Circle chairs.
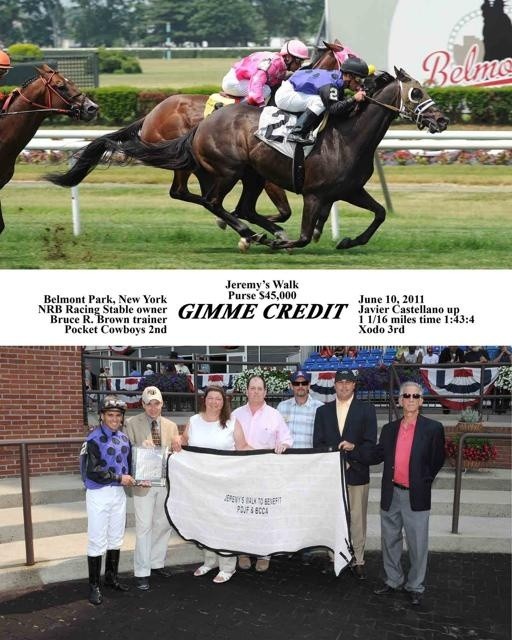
[371,348,383,355]
[383,354,394,362]
[431,346,442,352]
[342,356,355,364]
[311,365,323,370]
[303,365,312,371]
[311,350,320,359]
[369,355,381,362]
[386,347,396,355]
[329,357,340,363]
[338,363,350,371]
[363,363,376,368]
[324,365,337,370]
[358,349,370,356]
[351,364,359,369]
[356,356,367,362]
[317,358,328,364]
[305,357,315,364]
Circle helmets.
[339,56,369,78]
[97,392,127,412]
[0,51,12,68]
[279,39,309,60]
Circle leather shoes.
[134,576,150,590]
[353,564,368,579]
[151,567,173,579]
[373,581,398,595]
[402,586,422,606]
[238,557,252,569]
[255,558,270,571]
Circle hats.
[334,368,355,383]
[290,370,310,381]
[141,385,163,404]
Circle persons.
[129,365,139,374]
[278,370,327,573]
[104,367,110,378]
[145,364,154,375]
[418,348,438,367]
[226,376,292,573]
[346,348,357,356]
[96,367,105,374]
[466,347,490,364]
[179,387,247,583]
[79,398,150,605]
[338,382,446,607]
[1,53,13,110]
[217,39,311,107]
[320,347,334,357]
[439,346,463,415]
[274,56,367,146]
[129,385,182,591]
[176,357,190,373]
[193,357,210,373]
[315,368,377,578]
[493,347,512,412]
[394,347,422,368]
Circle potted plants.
[457,407,482,434]
[446,436,499,472]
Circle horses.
[104,64,451,256]
[39,38,378,243]
[0,64,100,236]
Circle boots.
[286,107,319,145]
[88,555,104,605]
[105,548,130,592]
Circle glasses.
[291,380,310,386]
[400,393,421,399]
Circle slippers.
[212,569,236,583]
[193,563,212,576]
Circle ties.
[151,420,161,447]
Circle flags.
[163,443,354,576]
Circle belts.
[392,481,410,491]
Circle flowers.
[234,366,293,396]
[494,365,512,393]
